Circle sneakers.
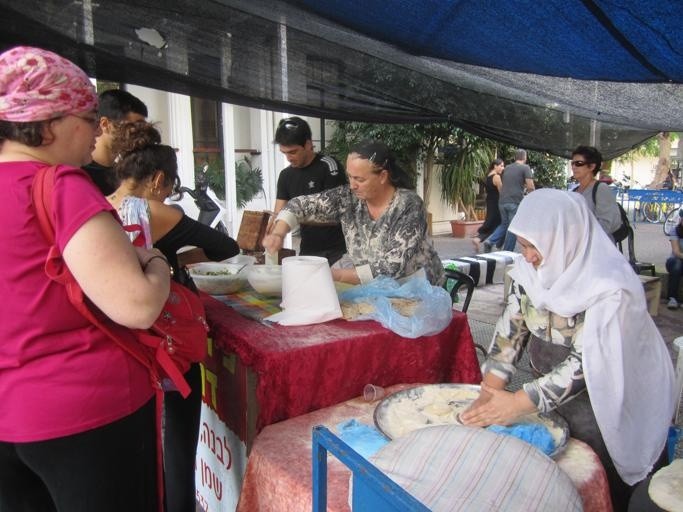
[666,295,679,309]
[471,236,481,254]
[483,239,492,253]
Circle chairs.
[443,268,488,358]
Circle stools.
[613,226,656,276]
[638,274,661,316]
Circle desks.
[235,381,612,512]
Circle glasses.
[278,116,310,139]
[570,159,590,168]
[69,112,103,128]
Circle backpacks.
[34,164,208,399]
[573,180,634,250]
[104,121,210,511]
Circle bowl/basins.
[245,264,283,298]
[186,261,248,294]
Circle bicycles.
[644,194,679,223]
[662,208,683,236]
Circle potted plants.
[193,156,268,236]
[439,135,494,237]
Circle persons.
[81,89,148,197]
[571,146,622,248]
[0,45,171,512]
[666,209,683,309]
[263,117,348,267]
[264,140,448,288]
[483,149,535,253]
[472,159,504,253]
[461,188,680,512]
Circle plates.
[373,382,570,460]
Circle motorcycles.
[170,162,229,238]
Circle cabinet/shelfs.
[147,279,485,511]
